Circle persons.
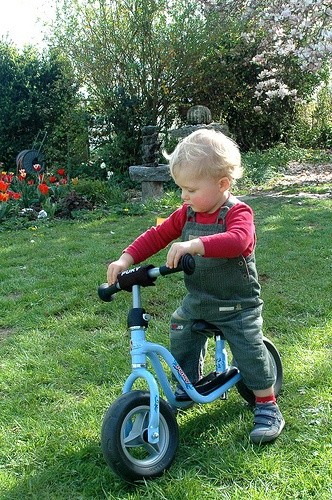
[105,127,287,443]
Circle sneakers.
[250,402,285,444]
[169,389,194,409]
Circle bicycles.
[96,252,284,485]
